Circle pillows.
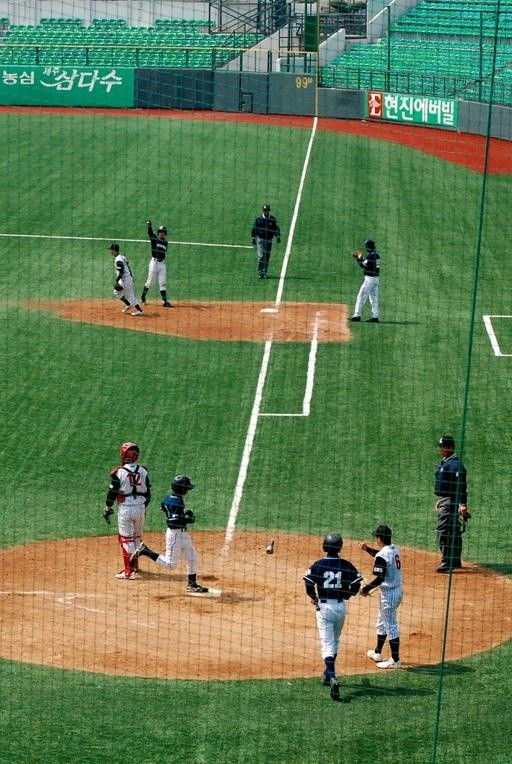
[323,532,345,553]
[121,442,140,465]
[157,225,167,235]
[365,239,375,248]
[172,475,197,490]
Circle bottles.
[107,243,120,252]
[262,204,271,210]
[438,435,455,446]
[372,525,394,538]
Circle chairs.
[367,319,380,323]
[366,650,383,659]
[322,675,329,681]
[122,305,135,314]
[186,584,208,592]
[163,302,172,306]
[436,562,452,572]
[131,311,145,316]
[454,559,461,565]
[116,572,136,578]
[264,274,270,278]
[350,316,362,322]
[131,544,146,562]
[330,678,339,700]
[257,274,263,279]
[141,298,147,306]
[376,658,398,666]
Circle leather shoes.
[252,238,258,246]
[103,509,112,526]
[276,236,282,244]
[182,514,196,523]
[185,510,193,515]
[113,282,122,290]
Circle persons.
[251,204,281,279]
[359,525,404,668]
[346,240,381,322]
[130,476,209,593]
[107,243,145,316]
[102,442,151,580]
[141,219,175,307]
[303,532,364,700]
[434,436,472,573]
